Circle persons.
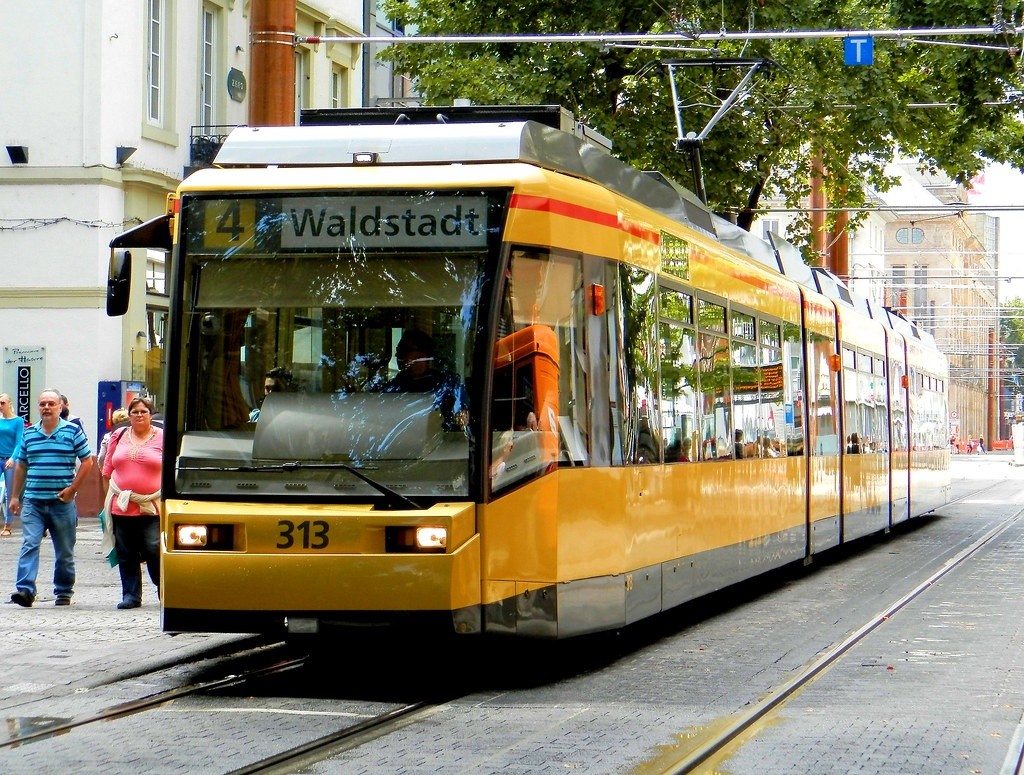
[43,395,83,537]
[948,432,1014,455]
[636,415,945,464]
[8,387,94,608]
[247,366,294,423]
[0,393,24,537]
[99,396,163,609]
[97,408,132,473]
[369,329,472,432]
[142,397,164,429]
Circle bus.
[101,55,955,643]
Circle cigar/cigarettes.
[13,506,20,514]
